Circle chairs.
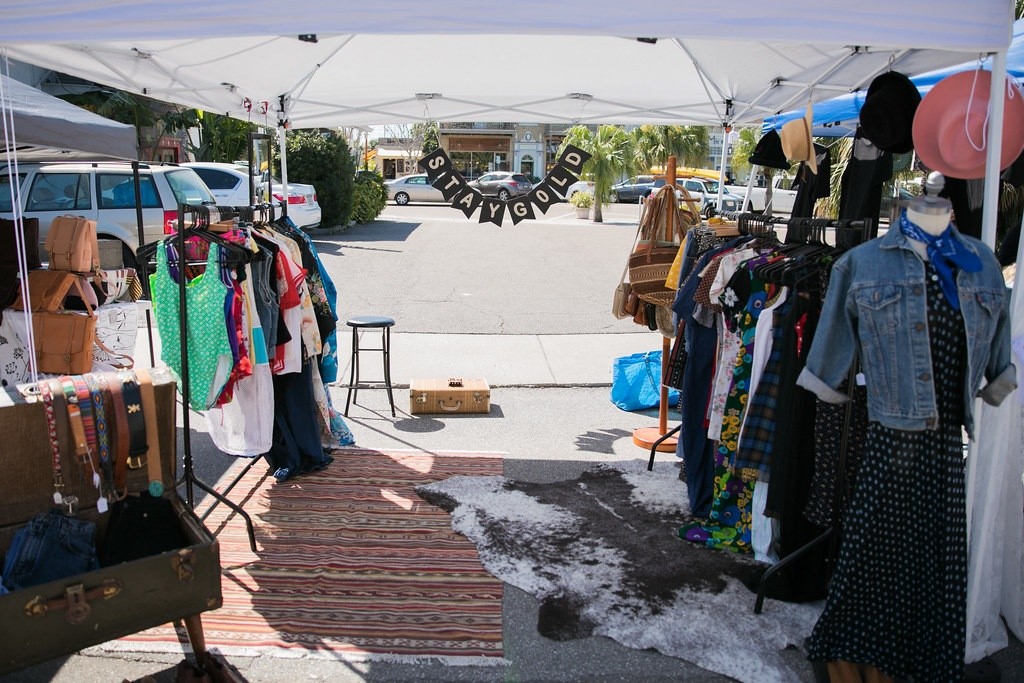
[32,188,61,211]
[64,185,85,208]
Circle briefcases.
[409,376,491,415]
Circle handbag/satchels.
[610,350,681,412]
[612,182,702,339]
[12,214,144,375]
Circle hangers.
[707,207,852,291]
[135,201,289,267]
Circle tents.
[740,9,1023,213]
[0,64,157,371]
[0,1,1017,566]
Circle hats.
[781,101,818,175]
[748,129,791,170]
[860,71,922,154]
[912,69,1024,179]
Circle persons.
[797,196,1019,683]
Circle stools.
[344,316,396,417]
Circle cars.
[879,185,915,217]
[608,175,656,203]
[384,174,482,205]
[533,174,596,198]
[102,162,322,230]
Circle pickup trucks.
[723,176,798,213]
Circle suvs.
[651,176,746,219]
[0,161,220,269]
[466,172,532,201]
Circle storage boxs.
[410,378,490,414]
[0,368,222,666]
[98,239,123,270]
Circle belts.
[31,365,164,515]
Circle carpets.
[78,445,510,666]
[416,469,826,683]
